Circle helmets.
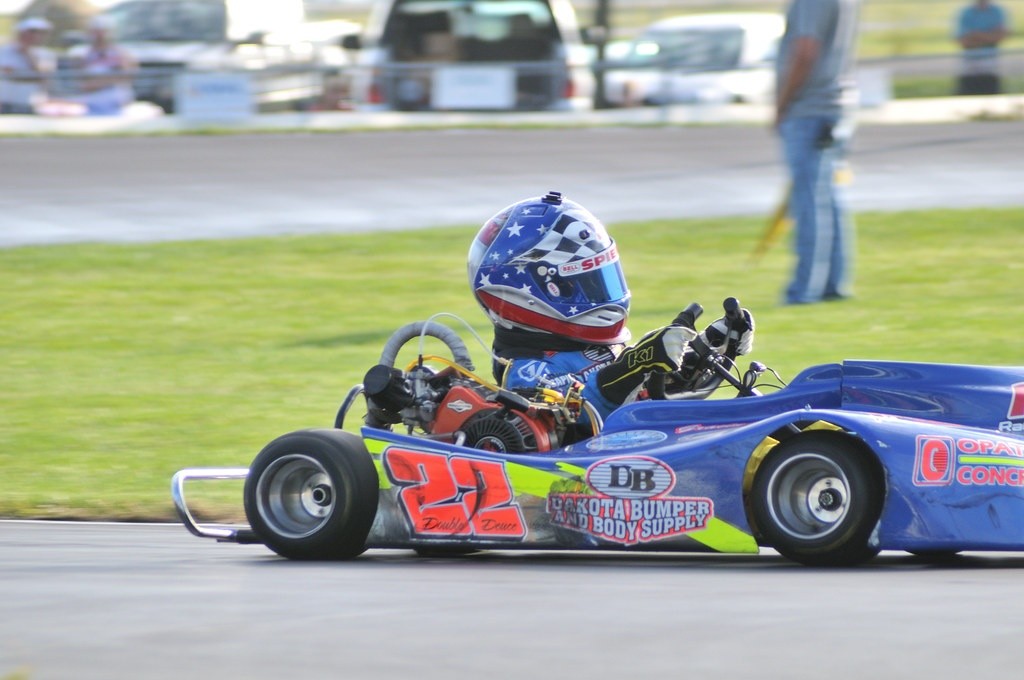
[466,191,632,346]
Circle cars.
[603,14,886,106]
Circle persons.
[772,1,864,308]
[0,16,141,120]
[952,3,1010,96]
[467,192,754,449]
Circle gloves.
[598,325,695,406]
[668,307,757,356]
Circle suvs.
[343,0,601,113]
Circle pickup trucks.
[90,2,361,114]
